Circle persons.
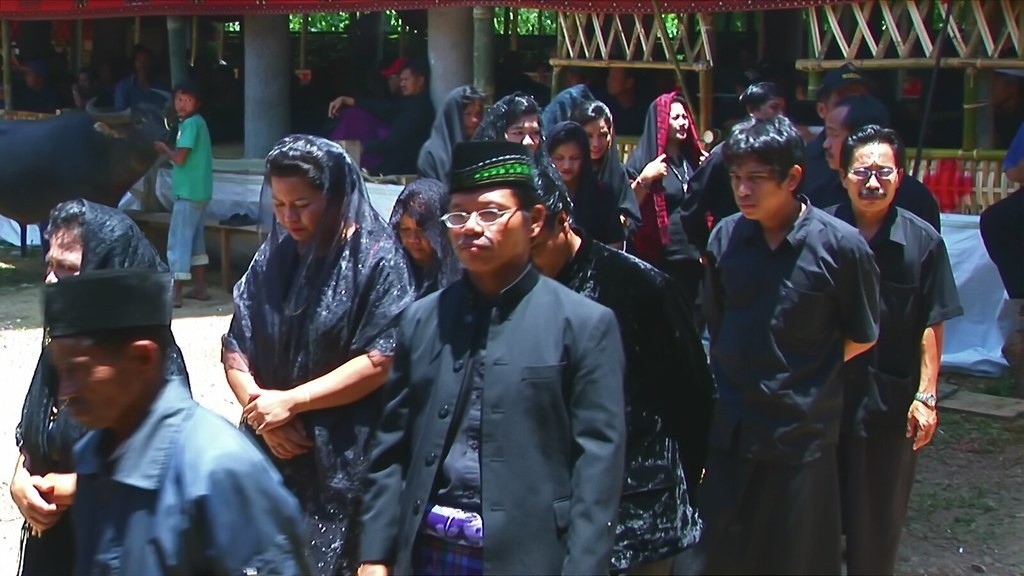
[0,17,1024,576]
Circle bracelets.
[636,177,650,194]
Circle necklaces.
[666,158,689,193]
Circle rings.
[277,446,283,451]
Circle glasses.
[438,207,518,228]
[848,167,897,180]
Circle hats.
[42,271,175,337]
[448,141,538,195]
[824,61,872,89]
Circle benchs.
[122,210,272,293]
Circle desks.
[934,211,1011,380]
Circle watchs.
[915,391,936,409]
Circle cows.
[0,88,178,259]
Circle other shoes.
[181,289,212,301]
